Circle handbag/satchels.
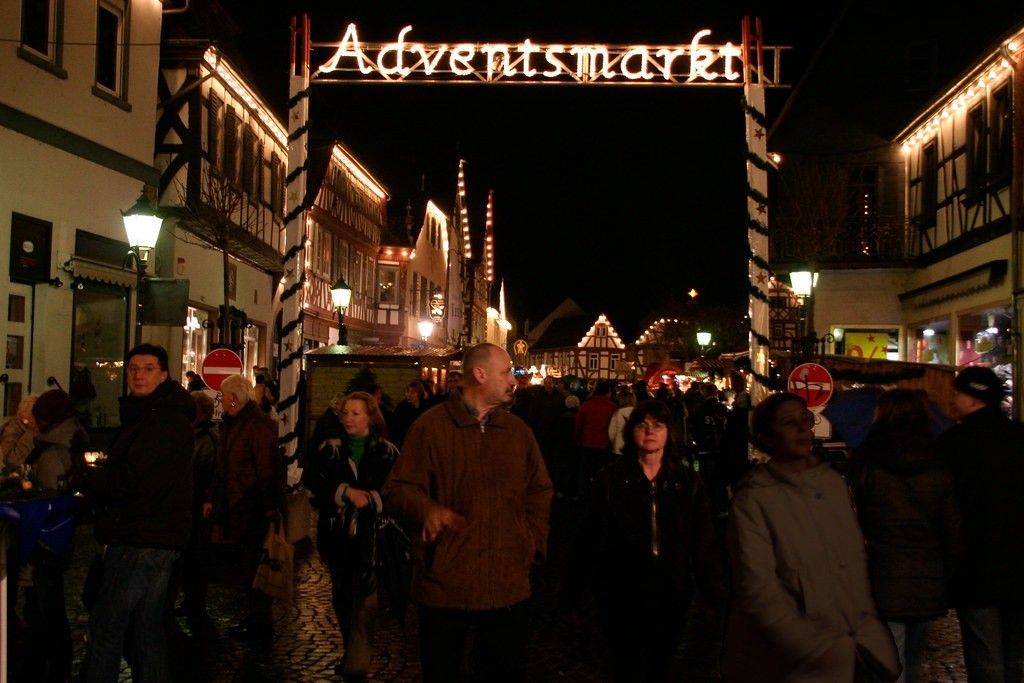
[251,512,294,603]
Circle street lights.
[789,259,820,362]
[329,276,352,346]
[119,194,165,348]
[696,326,712,372]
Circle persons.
[298,366,1024,683]
[0,367,292,683]
[729,392,903,682]
[378,342,554,683]
[76,343,196,683]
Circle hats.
[952,367,1003,406]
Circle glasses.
[634,420,666,433]
[128,365,162,374]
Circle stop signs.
[202,348,244,392]
[788,363,833,408]
